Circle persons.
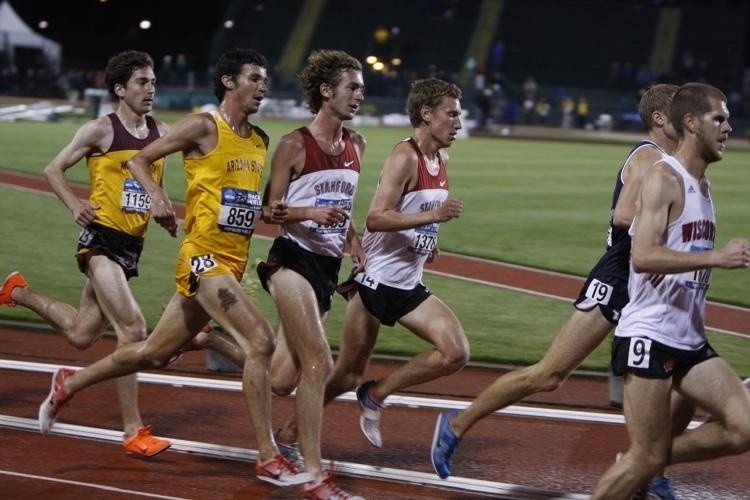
[588,84,750,500]
[428,81,709,500]
[166,45,367,500]
[0,51,175,460]
[273,77,468,473]
[35,45,316,487]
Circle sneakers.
[647,476,676,499]
[38,367,77,439]
[0,270,29,310]
[355,379,383,449]
[168,321,214,368]
[255,452,315,487]
[293,471,366,500]
[122,424,172,458]
[273,426,305,473]
[430,411,463,480]
